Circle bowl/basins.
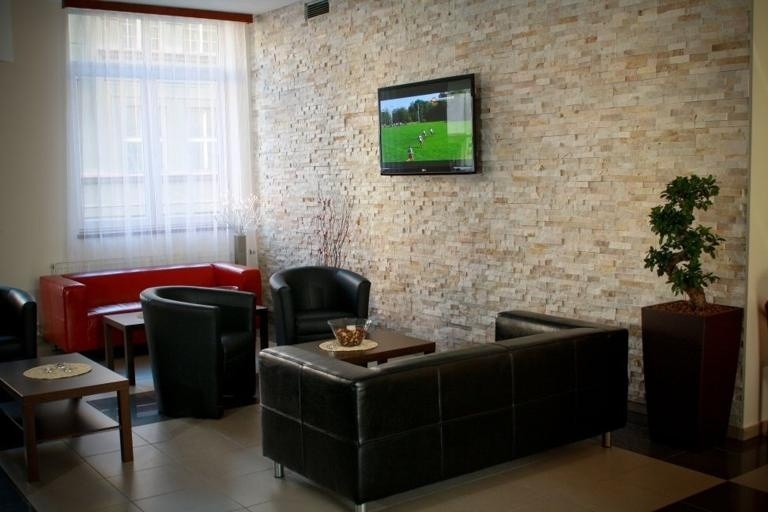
[329,318,373,347]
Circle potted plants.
[640,170,744,450]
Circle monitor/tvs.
[377,73,481,175]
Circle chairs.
[269,265,371,346]
[0,286,37,362]
[139,285,257,421]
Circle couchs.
[39,261,263,353]
[0,465,38,512]
[258,310,629,512]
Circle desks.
[289,330,436,368]
[0,352,134,483]
[102,305,269,387]
